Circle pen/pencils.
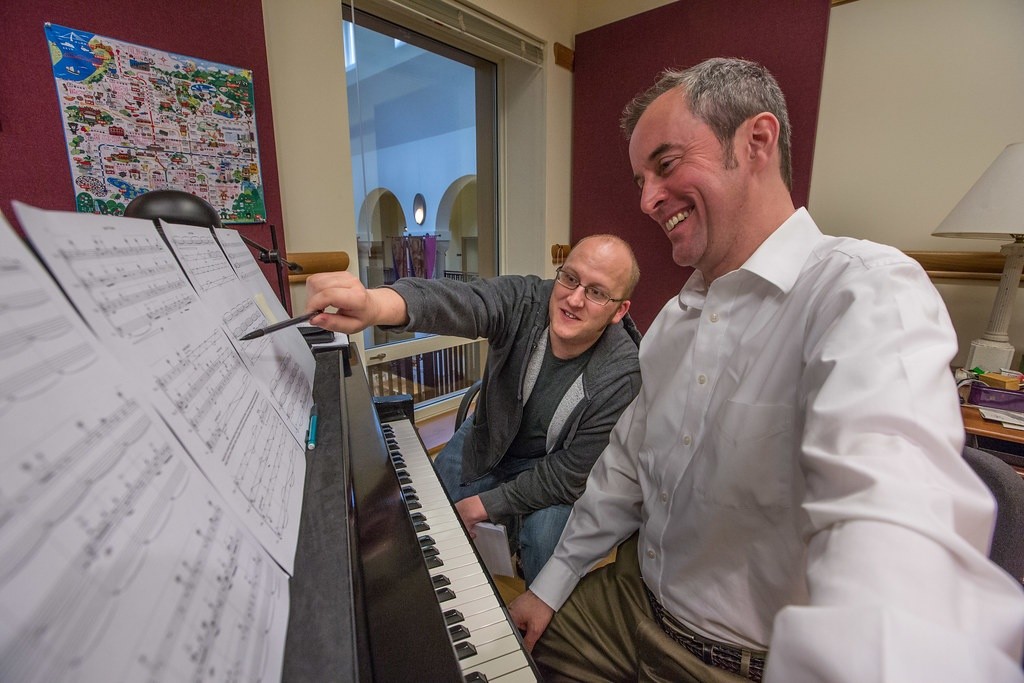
[238,309,324,342]
[304,402,319,451]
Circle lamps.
[930,143,1024,380]
[123,189,303,316]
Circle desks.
[958,386,1024,478]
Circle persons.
[306,234,643,591]
[507,57,1024,683]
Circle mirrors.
[413,193,426,225]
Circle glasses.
[556,266,626,306]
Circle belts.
[642,579,765,683]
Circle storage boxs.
[969,386,1024,413]
[980,369,1022,390]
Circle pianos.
[0,230,548,683]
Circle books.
[979,408,1024,430]
